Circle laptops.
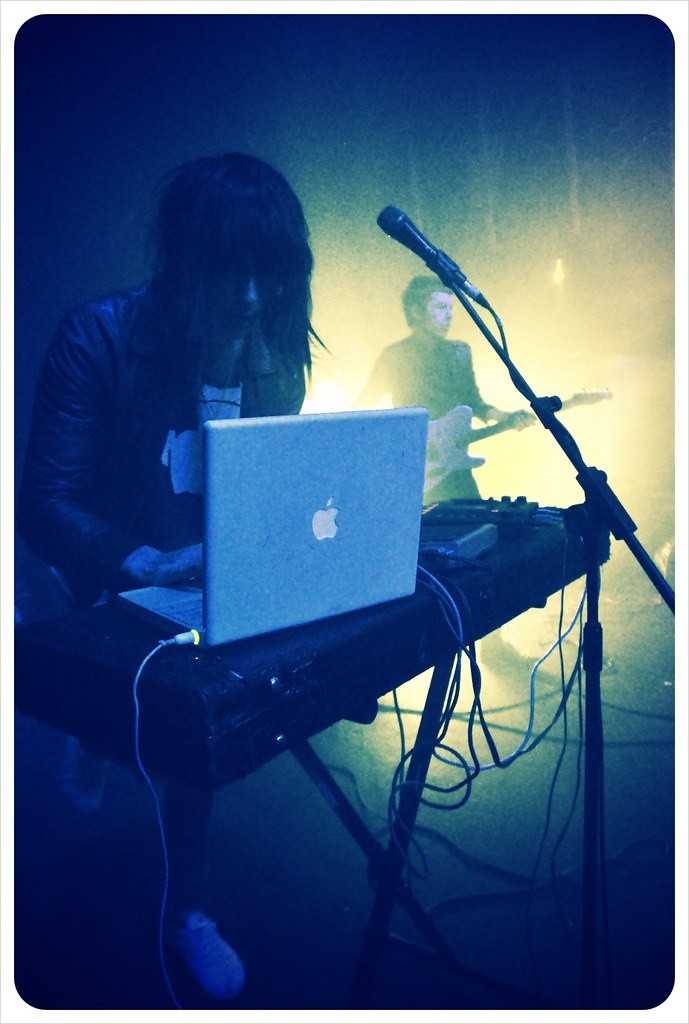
[116,407,428,649]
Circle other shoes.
[483,643,539,670]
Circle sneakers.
[178,911,244,998]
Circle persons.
[16,149,333,1003]
[364,273,533,507]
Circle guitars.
[420,392,612,493]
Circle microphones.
[377,205,490,311]
